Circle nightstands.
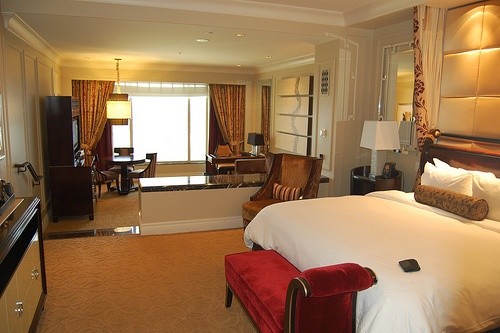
[351,165,403,196]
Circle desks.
[206,153,265,175]
[101,155,145,195]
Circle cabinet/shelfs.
[44,96,97,223]
[0,197,47,333]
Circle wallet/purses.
[399,259,420,272]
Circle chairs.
[127,153,157,191]
[109,148,134,190]
[87,155,120,198]
[241,153,324,230]
[214,143,237,175]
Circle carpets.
[34,182,260,333]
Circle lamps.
[246,133,264,156]
[360,117,400,176]
[106,58,131,125]
[398,120,410,145]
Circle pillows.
[272,183,302,202]
[414,158,500,221]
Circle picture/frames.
[397,102,413,126]
[0,94,6,159]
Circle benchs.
[225,249,377,333]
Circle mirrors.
[376,41,416,154]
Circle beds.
[241,129,500,333]
